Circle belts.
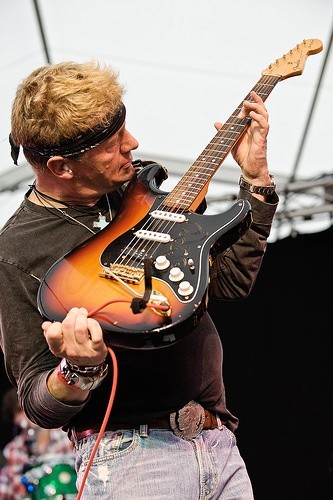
[69,398,227,445]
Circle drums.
[23,453,79,500]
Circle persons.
[0,389,71,500]
[1,63,279,500]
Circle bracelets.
[56,358,109,391]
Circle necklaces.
[29,180,112,234]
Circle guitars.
[37,37,323,349]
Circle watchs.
[238,174,277,196]
